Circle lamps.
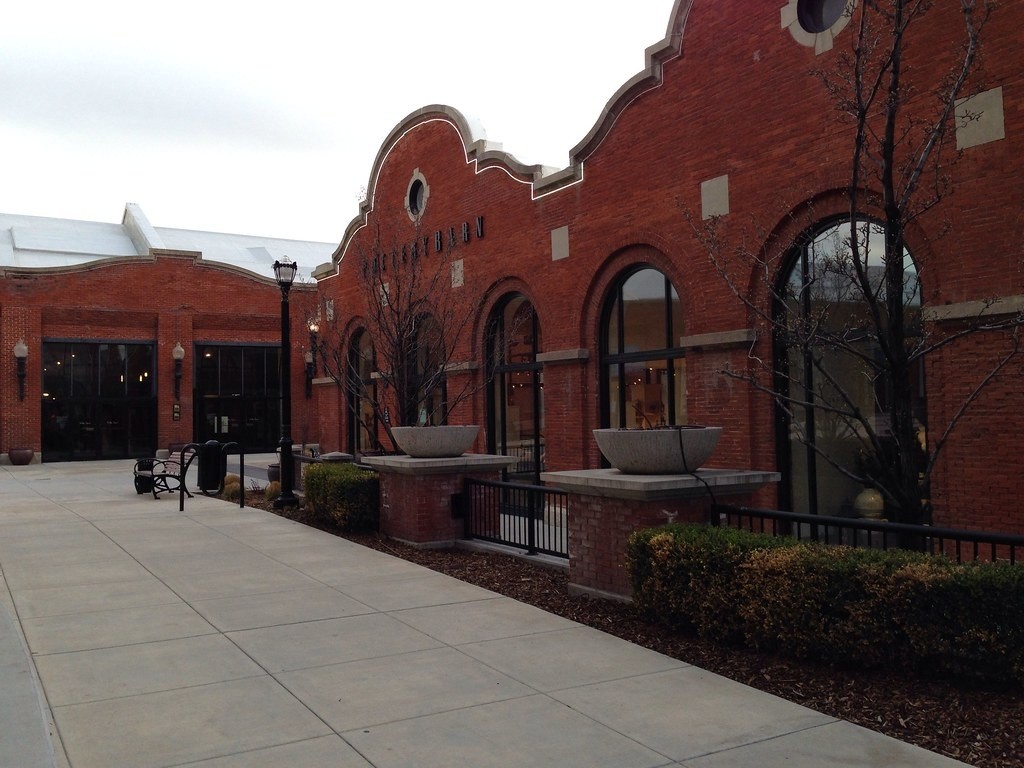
[14,338,28,401]
[305,351,314,400]
[307,317,319,375]
[172,342,185,400]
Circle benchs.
[134,451,196,499]
